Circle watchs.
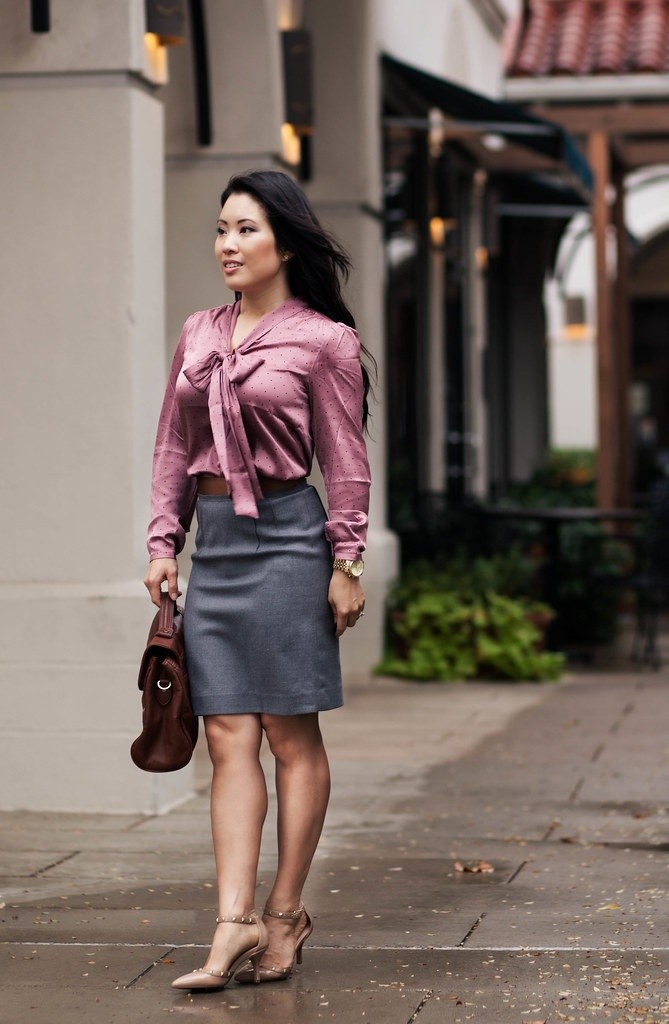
[332,559,364,578]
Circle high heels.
[171,914,269,989]
[234,900,313,985]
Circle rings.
[356,611,365,619]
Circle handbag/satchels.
[130,592,199,773]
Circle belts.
[197,475,295,497]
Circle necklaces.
[146,169,378,994]
